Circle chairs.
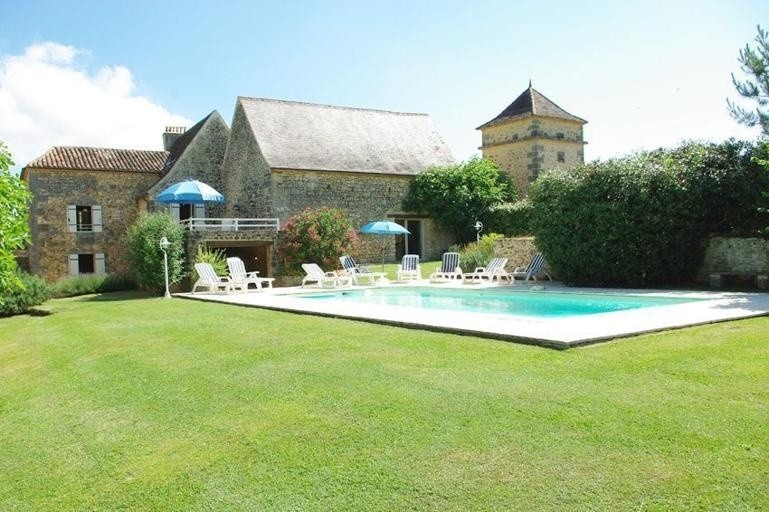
[228,256,275,293]
[300,262,351,290]
[339,255,387,287]
[191,263,236,295]
[469,257,510,284]
[397,255,421,282]
[431,252,464,281]
[508,252,553,285]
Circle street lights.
[158,236,171,298]
[473,220,484,244]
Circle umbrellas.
[356,220,411,277]
[154,180,225,231]
